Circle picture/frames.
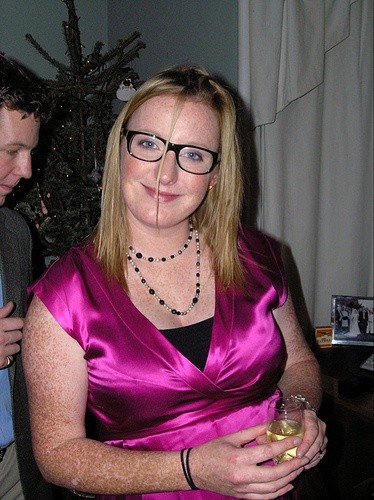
[330,295,374,345]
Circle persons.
[0,44,35,500]
[20,67,328,500]
[334,301,374,338]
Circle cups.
[265,397,305,465]
[315,326,333,348]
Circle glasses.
[120,128,221,175]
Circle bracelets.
[181,448,200,490]
[280,393,317,413]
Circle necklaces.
[126,222,202,316]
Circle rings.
[7,357,11,366]
